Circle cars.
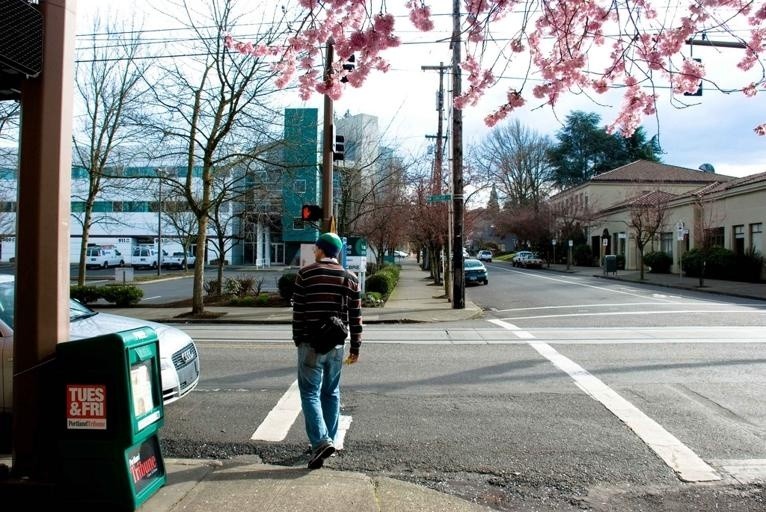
[0,274,200,413]
[463,247,493,286]
[512,251,543,268]
[384,250,408,259]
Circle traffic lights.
[302,205,322,221]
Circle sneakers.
[308,442,336,469]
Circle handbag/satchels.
[309,316,348,355]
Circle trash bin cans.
[604,255,617,276]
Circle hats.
[316,232,343,257]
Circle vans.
[131,244,168,270]
[85,245,124,270]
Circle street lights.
[462,184,492,246]
[155,168,170,276]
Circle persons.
[278,273,299,308]
[290,233,362,470]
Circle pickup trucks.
[162,251,197,270]
